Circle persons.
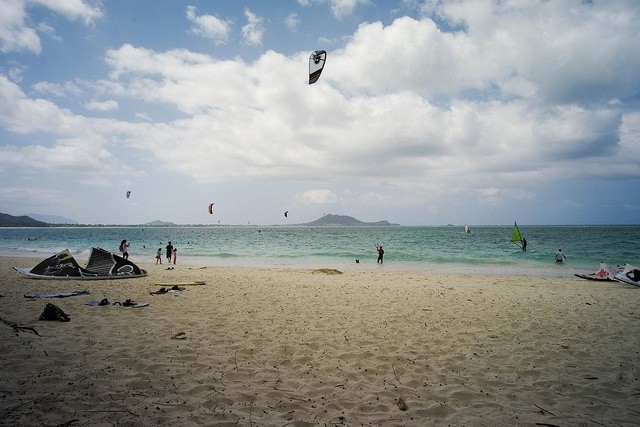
[521,237,527,251]
[173,248,177,264]
[120,239,130,260]
[375,243,385,264]
[165,241,173,262]
[155,247,164,264]
[554,248,566,263]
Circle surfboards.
[86,300,149,307]
[155,280,204,286]
[150,286,185,295]
